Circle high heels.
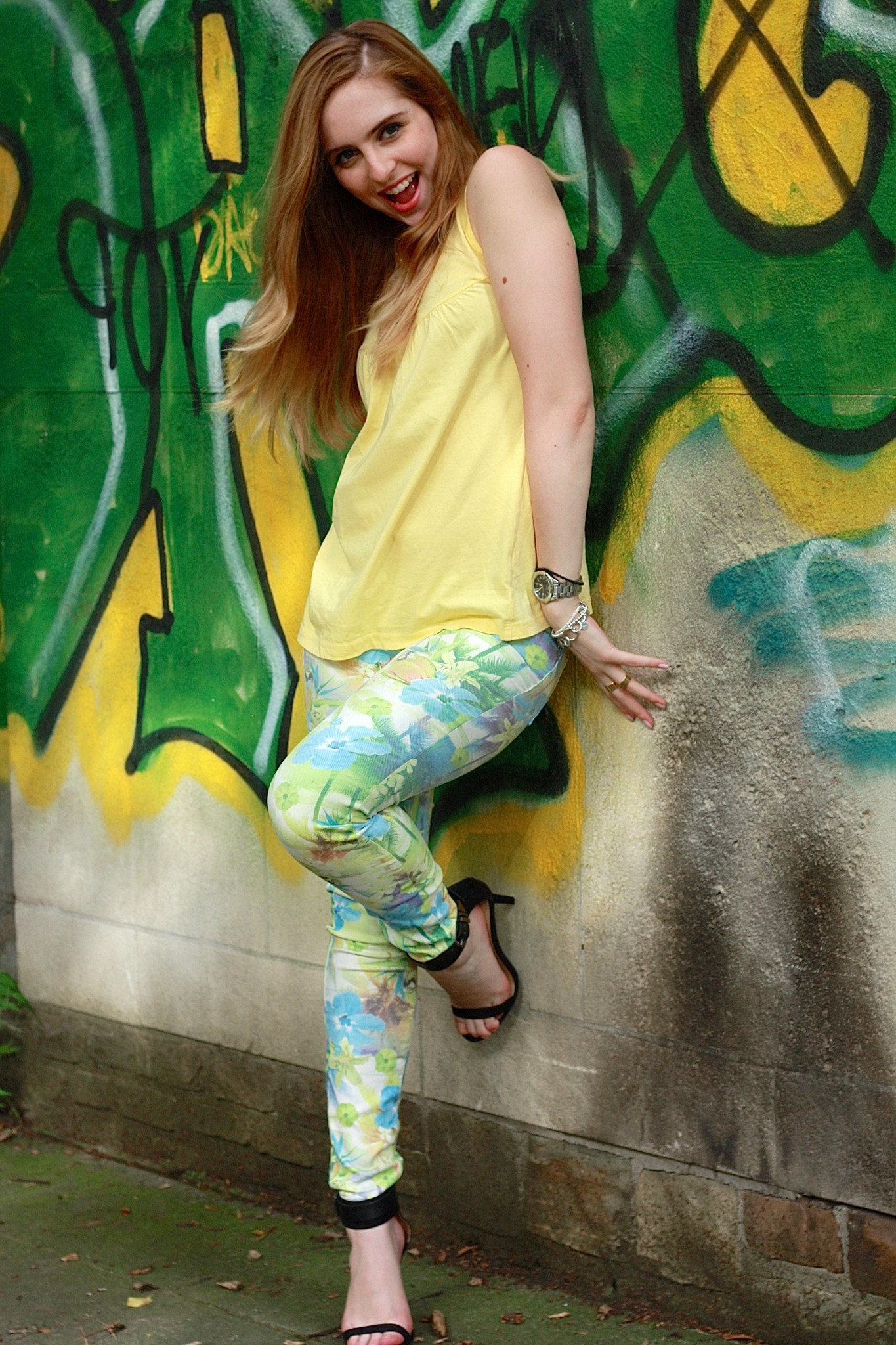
[336,1186,413,1345]
[404,877,521,1041]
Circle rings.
[604,681,618,694]
[616,671,632,690]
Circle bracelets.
[551,601,590,651]
[534,566,585,586]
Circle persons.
[210,19,670,1345]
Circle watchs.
[532,571,582,604]
